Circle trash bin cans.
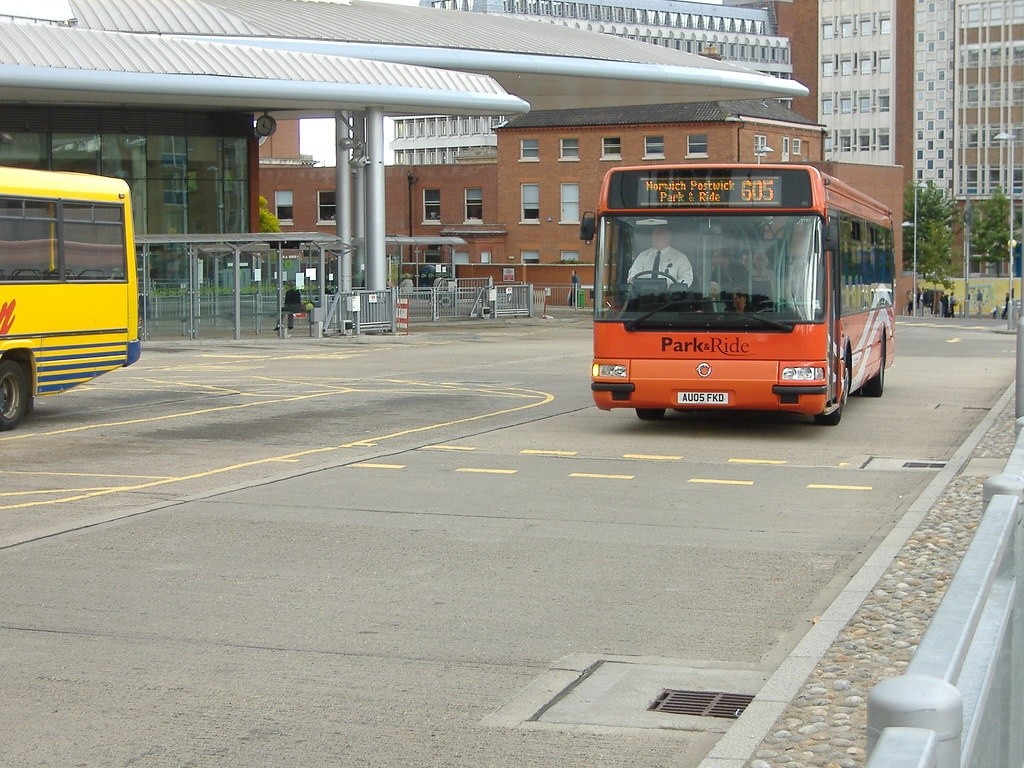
[440,278,457,307]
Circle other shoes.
[273,327,279,331]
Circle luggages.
[577,285,586,308]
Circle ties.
[652,251,661,278]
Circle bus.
[0,164,141,431]
[578,162,897,427]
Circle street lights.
[755,146,775,164]
[901,182,929,317]
[993,132,1018,331]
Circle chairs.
[748,274,771,297]
[0,268,107,281]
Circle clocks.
[256,111,277,137]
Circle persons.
[907,285,960,320]
[568,270,580,307]
[708,243,780,317]
[273,284,301,331]
[628,223,693,295]
[1000,291,1010,320]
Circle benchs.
[146,310,312,340]
